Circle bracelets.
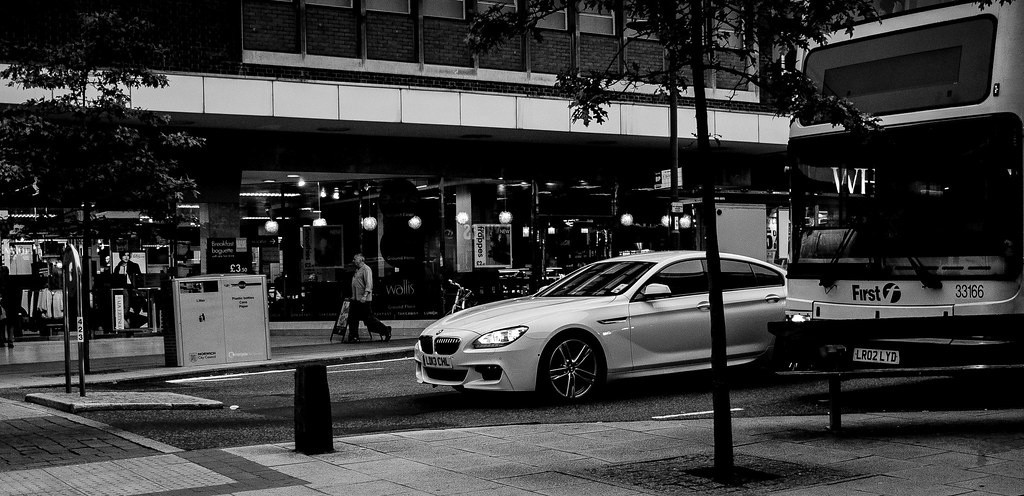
[363,295,367,297]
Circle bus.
[782,0,1023,367]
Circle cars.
[413,249,789,404]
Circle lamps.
[265,183,692,234]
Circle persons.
[490,227,508,265]
[115,252,141,274]
[343,252,391,343]
[0,265,22,347]
[315,236,336,267]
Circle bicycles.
[445,278,474,316]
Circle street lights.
[629,18,679,251]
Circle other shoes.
[0,343,6,347]
[344,336,360,343]
[8,344,14,348]
[383,326,392,341]
